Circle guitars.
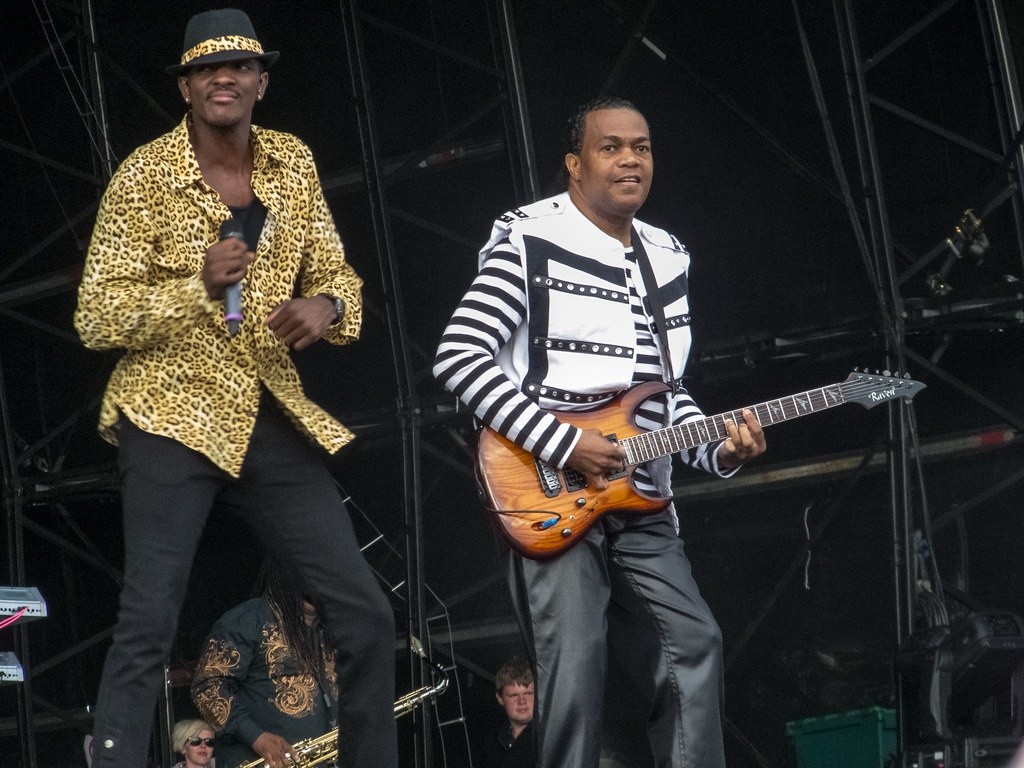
[473,364,930,564]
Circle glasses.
[184,737,215,747]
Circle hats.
[168,9,279,71]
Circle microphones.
[218,219,246,338]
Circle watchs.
[319,293,343,325]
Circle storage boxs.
[789,706,898,768]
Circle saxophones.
[229,658,453,768]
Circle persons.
[431,98,767,768]
[74,10,401,768]
[171,548,535,768]
